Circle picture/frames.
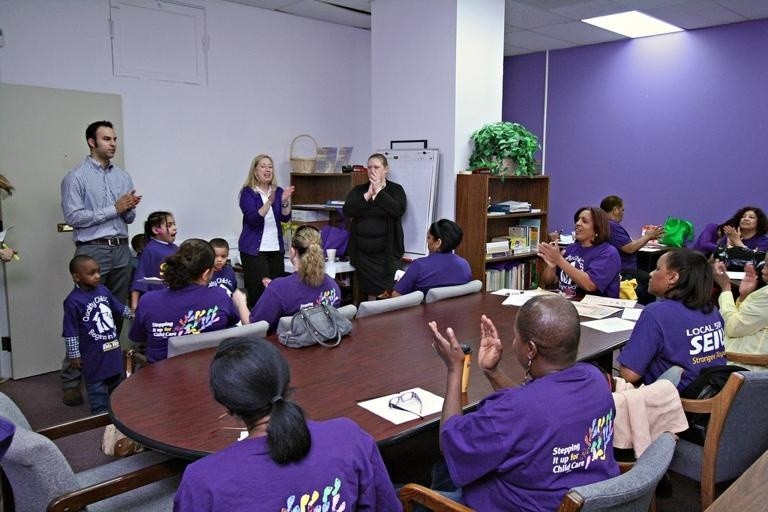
[106,0,208,85]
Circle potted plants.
[470,122,537,175]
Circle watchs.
[281,201,289,207]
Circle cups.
[326,248,336,264]
[459,344,473,393]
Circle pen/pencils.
[1,239,21,262]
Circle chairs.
[427,280,482,302]
[276,305,358,327]
[360,292,424,317]
[398,373,678,510]
[0,392,202,512]
[658,370,768,508]
[125,320,270,382]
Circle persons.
[62,255,133,415]
[600,196,666,271]
[130,233,151,309]
[238,155,295,311]
[617,249,726,393]
[206,238,237,293]
[232,227,341,337]
[61,120,142,407]
[342,153,407,301]
[172,336,404,512]
[392,220,471,297]
[127,238,241,365]
[537,206,620,299]
[128,234,145,311]
[0,173,18,262]
[394,295,621,511]
[717,207,768,259]
[691,218,730,261]
[716,254,768,370]
[131,211,179,316]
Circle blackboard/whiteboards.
[375,148,438,260]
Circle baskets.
[291,134,318,173]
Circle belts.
[78,238,129,247]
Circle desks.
[551,234,678,272]
[106,288,650,464]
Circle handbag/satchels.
[715,245,765,272]
[279,302,353,348]
[680,365,750,445]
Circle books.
[486,260,539,292]
[335,147,354,172]
[485,219,540,258]
[487,200,531,213]
[314,146,337,173]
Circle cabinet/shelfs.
[290,171,370,307]
[455,173,548,291]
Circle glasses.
[389,393,424,420]
[295,225,319,237]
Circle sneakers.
[62,387,83,405]
